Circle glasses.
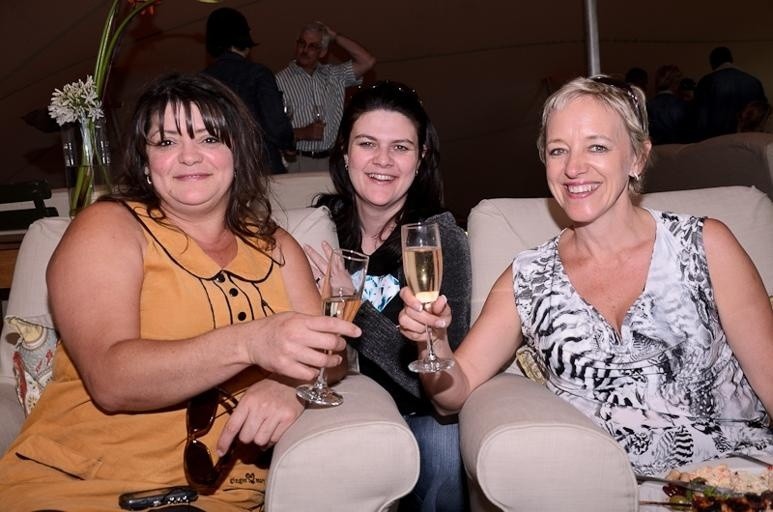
[295,38,321,51]
[589,77,644,131]
[183,385,240,486]
[369,79,424,110]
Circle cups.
[285,104,324,124]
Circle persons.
[0,60,361,511]
[625,45,772,146]
[297,85,474,511]
[199,8,296,177]
[396,72,773,479]
[273,20,376,172]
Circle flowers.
[47,0,154,214]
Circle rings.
[315,273,324,283]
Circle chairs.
[639,131,771,195]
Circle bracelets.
[331,32,342,45]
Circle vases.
[59,119,112,215]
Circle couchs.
[0,205,421,512]
[458,184,772,511]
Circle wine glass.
[401,223,456,372]
[295,247,368,406]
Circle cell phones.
[118,485,198,511]
